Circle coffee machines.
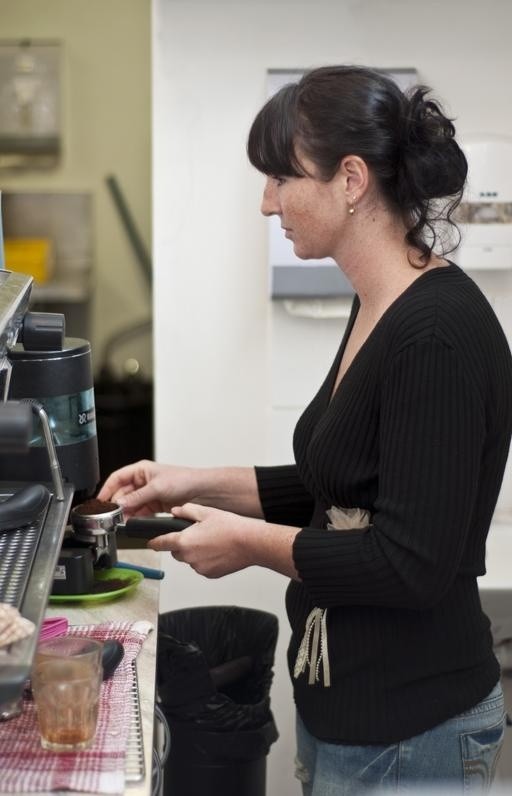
[1,265,191,722]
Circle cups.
[29,640,105,753]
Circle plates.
[49,565,145,606]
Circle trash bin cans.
[157,605,279,796]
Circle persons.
[97,65,512,796]
